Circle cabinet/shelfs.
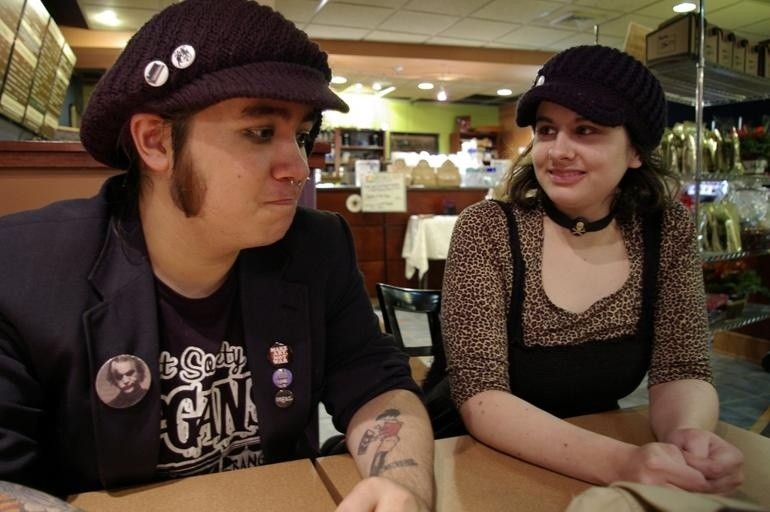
[450,130,502,159]
[643,0,770,336]
[335,127,386,178]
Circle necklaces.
[539,192,619,236]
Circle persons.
[420,45,745,496]
[0,0,440,511]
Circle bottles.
[465,166,497,187]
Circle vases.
[741,159,766,174]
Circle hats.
[513,44,670,162]
[76,0,353,171]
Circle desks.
[401,214,458,290]
[314,404,770,512]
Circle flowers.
[735,124,770,159]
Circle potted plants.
[712,269,763,320]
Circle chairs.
[375,282,442,370]
